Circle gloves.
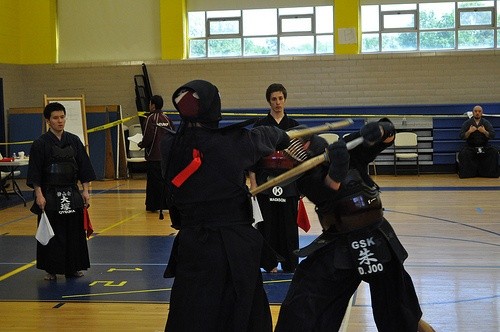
[329,140,350,176]
[360,122,382,142]
[276,129,291,151]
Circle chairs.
[393,132,419,177]
[126,140,146,181]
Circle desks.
[0,157,29,207]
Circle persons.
[457,106,500,179]
[137,95,177,212]
[274,117,435,332]
[162,80,291,332]
[26,102,97,282]
[246,83,302,273]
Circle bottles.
[12,153,16,162]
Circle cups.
[18,151,24,160]
